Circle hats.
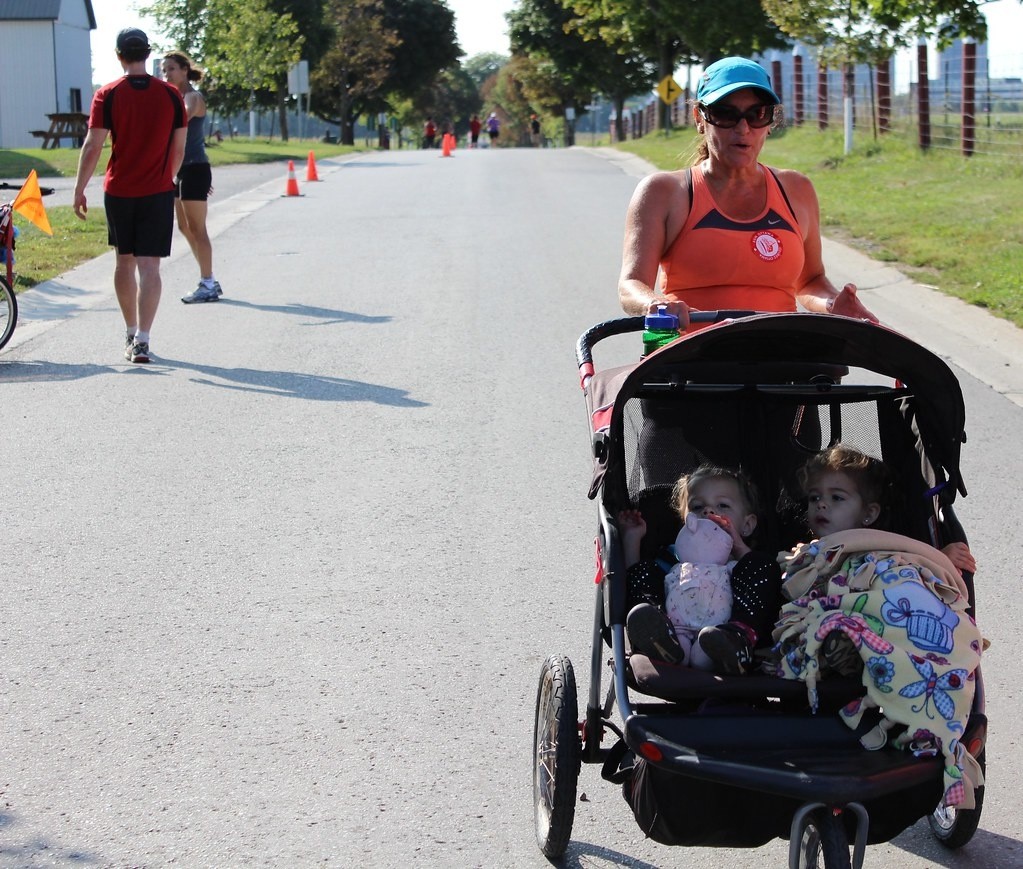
[697,57,780,105]
[117,26,149,50]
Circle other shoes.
[822,629,864,678]
[698,623,752,674]
[625,603,685,664]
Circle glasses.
[698,102,774,128]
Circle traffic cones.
[437,134,455,158]
[280,161,306,197]
[449,135,455,152]
[301,151,324,182]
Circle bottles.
[642,304,680,356]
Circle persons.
[470,116,481,148]
[426,116,437,149]
[487,112,500,148]
[161,52,223,304]
[618,468,782,687]
[617,56,879,450]
[803,444,977,751]
[529,114,540,147]
[72,27,188,363]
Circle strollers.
[532,304,989,869]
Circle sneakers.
[126,335,136,348]
[124,342,149,362]
[181,282,219,303]
[215,281,223,296]
